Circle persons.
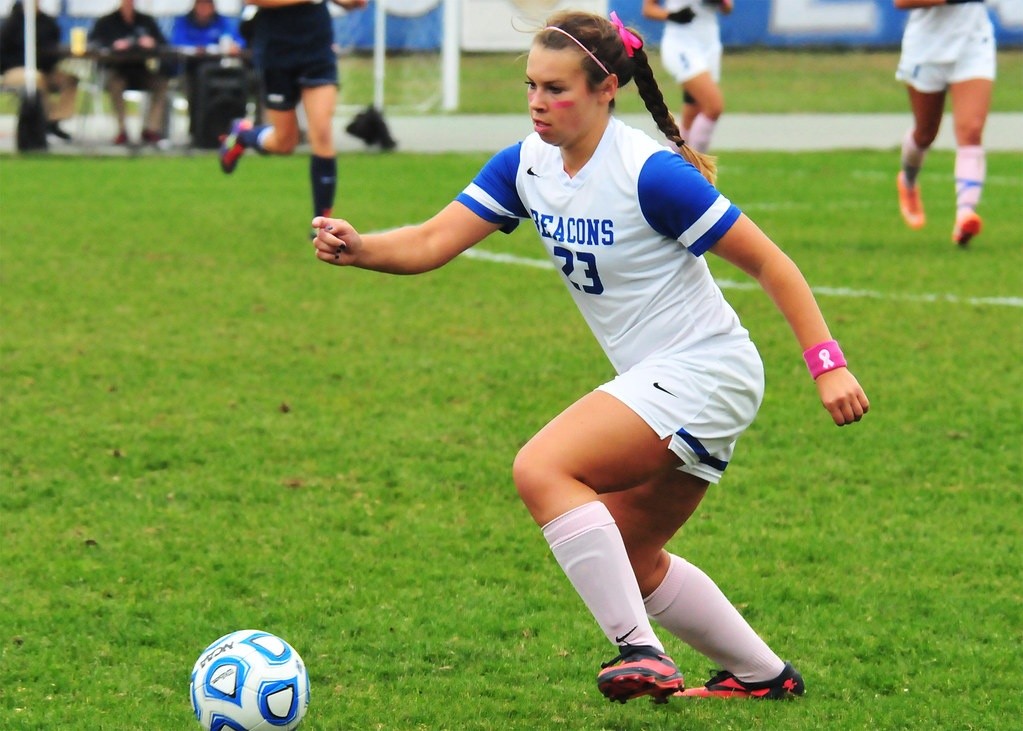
[312,10,869,705]
[0,0,367,241]
[890,0,998,247]
[642,0,733,157]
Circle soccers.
[189,629,311,731]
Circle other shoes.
[46,118,69,137]
[111,131,126,145]
[141,128,168,147]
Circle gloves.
[667,7,695,24]
[702,0,723,6]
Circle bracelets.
[802,340,847,379]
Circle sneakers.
[219,117,251,174]
[952,213,983,242]
[673,659,806,700]
[596,643,684,702]
[896,170,925,226]
